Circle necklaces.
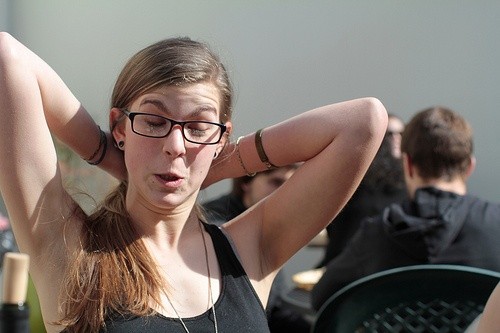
[130,216,218,333]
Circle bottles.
[0,301,30,333]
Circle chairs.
[309,265,500,333]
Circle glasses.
[119,108,226,145]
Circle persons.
[0,31,389,333]
[198,160,313,333]
[387,113,406,160]
[309,106,500,333]
[286,137,410,302]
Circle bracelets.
[235,135,257,177]
[255,127,281,169]
[83,125,107,166]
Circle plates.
[292,270,323,291]
